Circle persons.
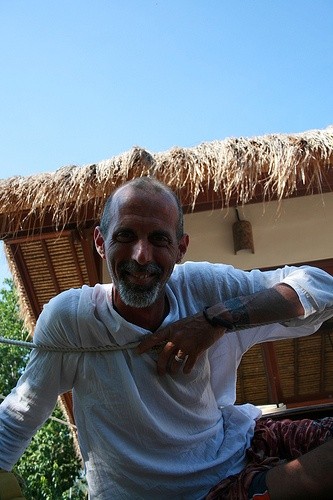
[0,172,332,500]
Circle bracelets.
[201,305,235,332]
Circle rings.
[174,354,185,363]
